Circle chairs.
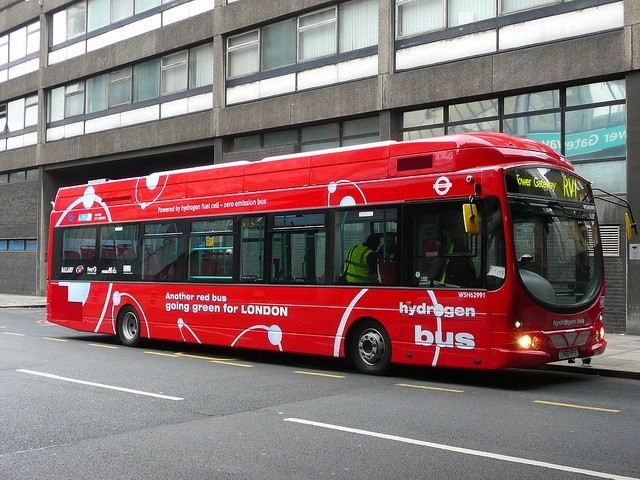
[80,245,96,260]
[101,245,116,260]
[62,251,80,260]
[117,244,134,259]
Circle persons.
[343,234,380,283]
[377,232,400,283]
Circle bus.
[46,132,637,375]
